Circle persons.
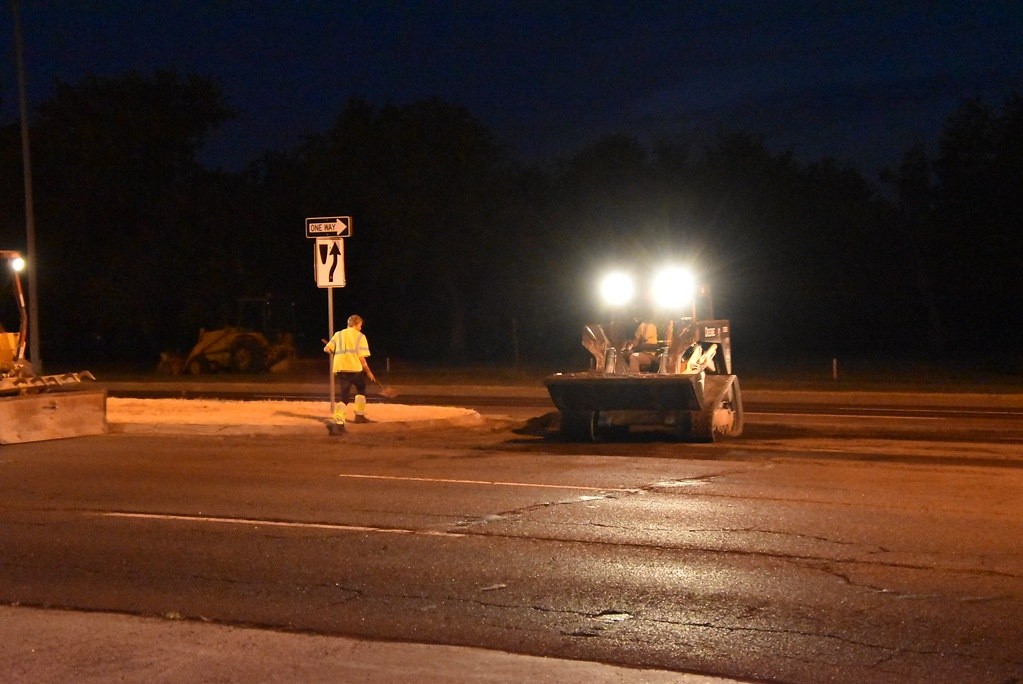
[323,315,375,436]
[621,307,658,371]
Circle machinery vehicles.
[0,249,108,445]
[157,297,298,380]
[542,266,741,442]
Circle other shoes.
[355,415,370,424]
[333,424,349,434]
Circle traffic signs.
[306,216,351,238]
